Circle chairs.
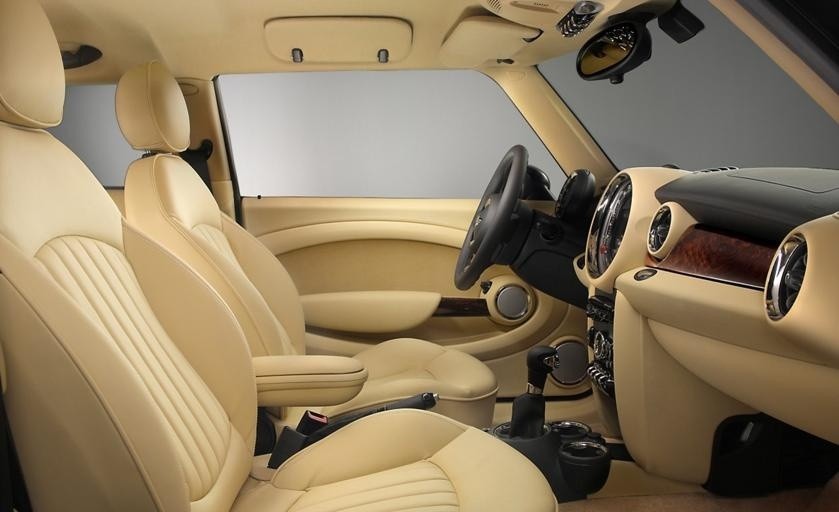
[114,61,499,434]
[0,2,562,510]
